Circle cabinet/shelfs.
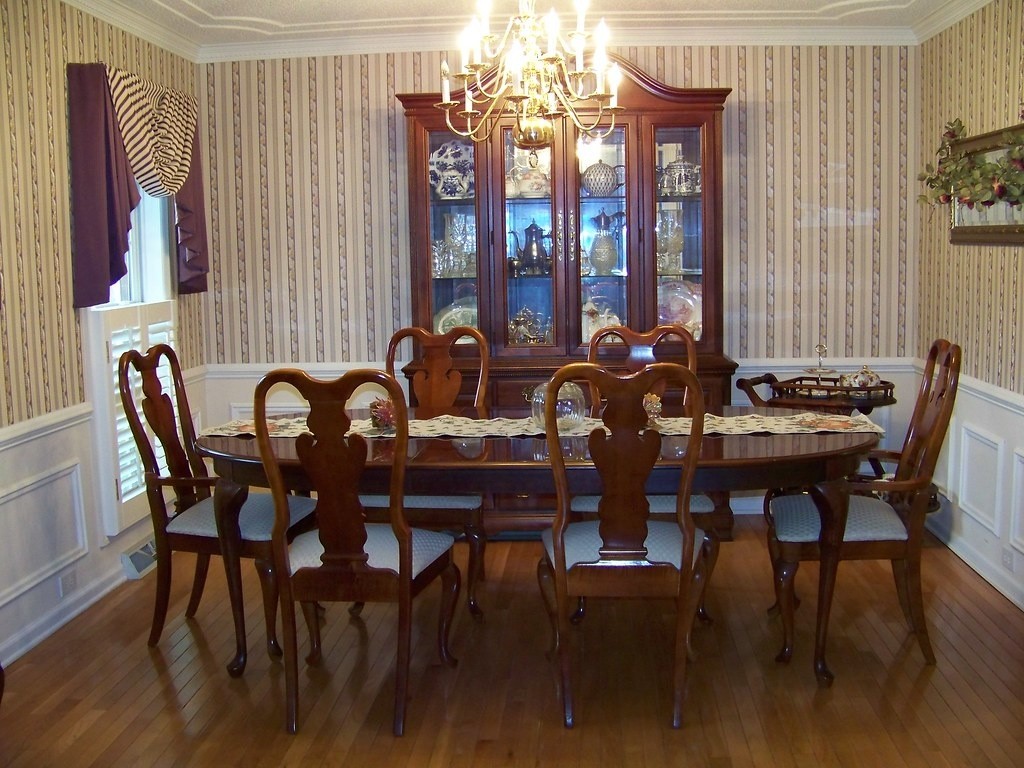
[393,47,739,542]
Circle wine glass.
[445,214,466,275]
[656,209,685,272]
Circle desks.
[191,407,883,690]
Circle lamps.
[434,1,628,169]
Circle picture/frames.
[947,122,1024,246]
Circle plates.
[657,280,702,341]
[428,139,475,199]
[434,296,477,344]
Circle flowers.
[915,119,1024,213]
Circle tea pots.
[510,166,551,198]
[666,155,702,194]
[580,159,626,196]
[505,172,520,197]
[677,177,678,177]
[588,207,617,276]
[509,218,553,275]
[582,298,609,343]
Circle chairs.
[117,341,317,680]
[567,324,723,627]
[345,325,491,620]
[762,339,962,665]
[534,362,723,730]
[253,368,463,740]
